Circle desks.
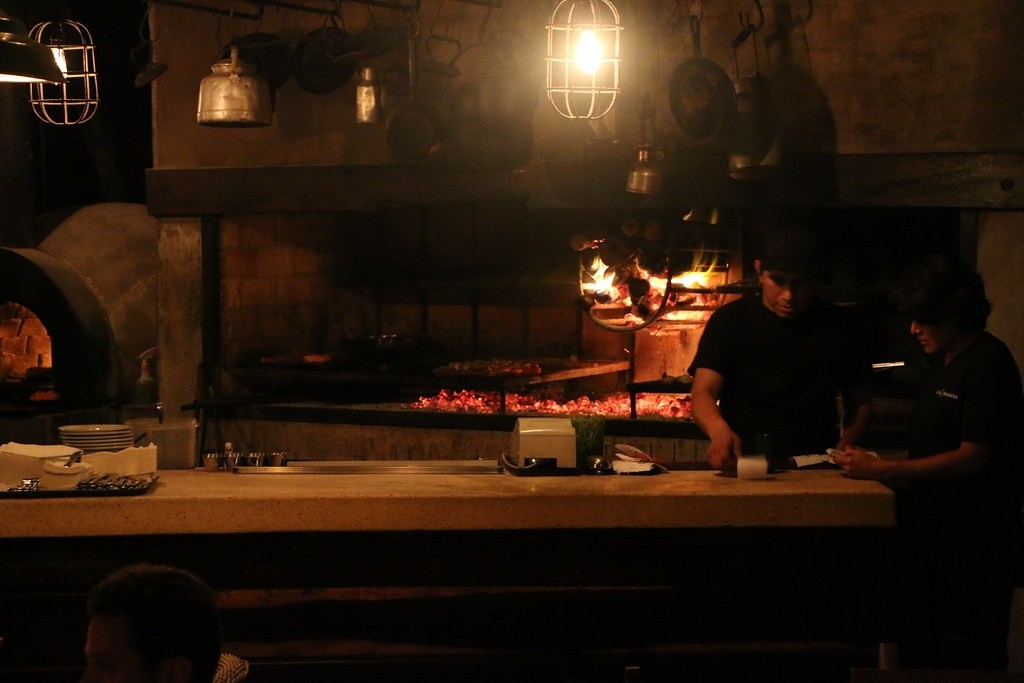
[0,461,900,669]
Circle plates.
[58,424,134,454]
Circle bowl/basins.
[0,442,83,488]
[43,460,92,490]
[80,446,157,477]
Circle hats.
[760,222,823,280]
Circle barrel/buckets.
[125,418,198,468]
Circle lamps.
[545,0,623,119]
[0,8,67,83]
[29,20,99,124]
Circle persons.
[78,564,224,683]
[689,224,874,479]
[825,265,1024,671]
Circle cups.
[219,452,288,469]
[202,453,218,472]
